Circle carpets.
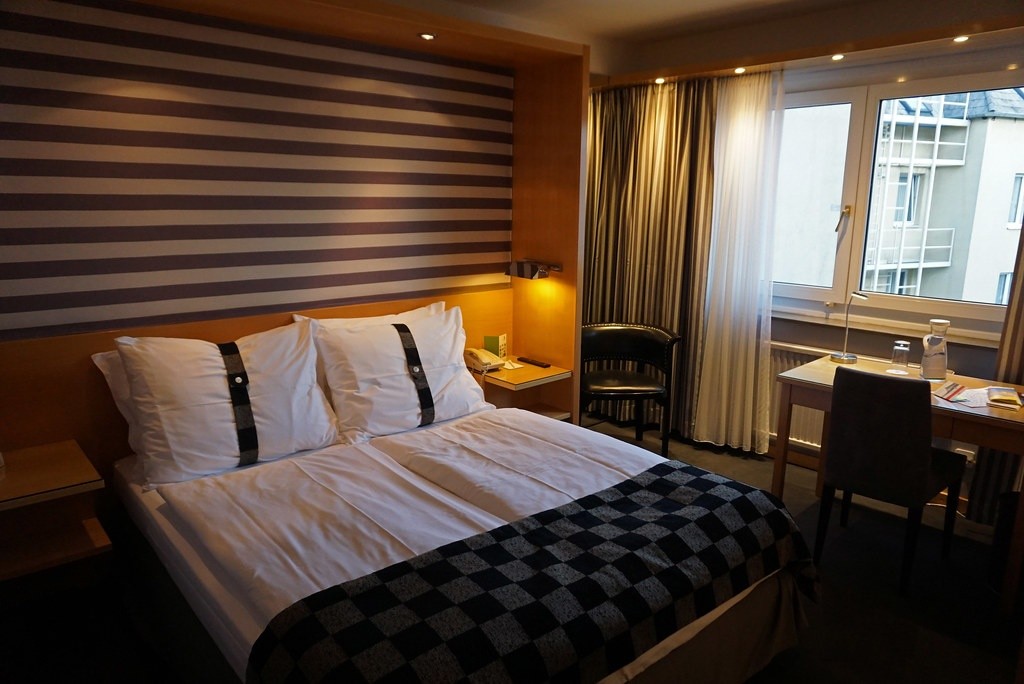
[829,514,1024,668]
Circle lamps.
[830,290,868,364]
[504,259,563,281]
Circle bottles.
[920,319,951,381]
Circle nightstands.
[0,439,113,582]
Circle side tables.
[467,354,572,420]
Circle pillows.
[114,317,348,491]
[92,349,147,485]
[293,301,446,403]
[315,306,485,444]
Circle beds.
[113,424,802,684]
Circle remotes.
[517,356,551,368]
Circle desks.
[769,351,1024,586]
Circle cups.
[889,340,910,375]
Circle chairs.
[812,366,966,599]
[581,322,680,458]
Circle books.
[986,385,1024,412]
[932,381,970,402]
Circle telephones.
[464,348,505,371]
